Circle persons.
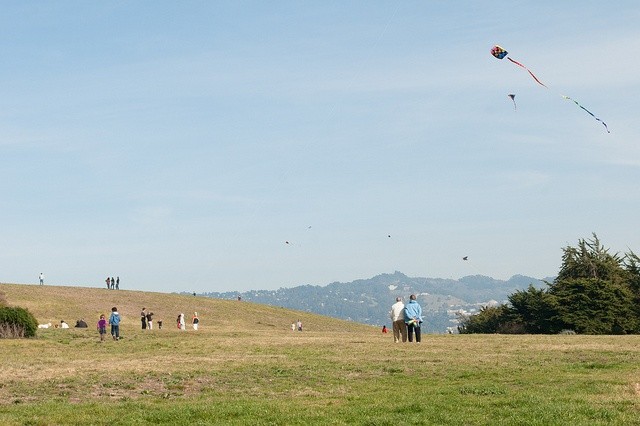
[145,311,154,330]
[140,307,146,329]
[75,320,79,327]
[116,276,119,289]
[111,277,114,289]
[79,318,88,327]
[290,320,295,331]
[180,313,185,329]
[382,325,388,332]
[61,320,69,328]
[404,294,423,344]
[97,314,107,340]
[192,311,199,330]
[296,319,302,331]
[177,315,181,329]
[390,297,407,343]
[105,277,110,288]
[109,307,121,340]
[39,273,43,285]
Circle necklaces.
[157,319,162,329]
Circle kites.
[509,94,518,106]
[491,44,611,134]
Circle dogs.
[55,324,59,327]
[38,322,51,328]
